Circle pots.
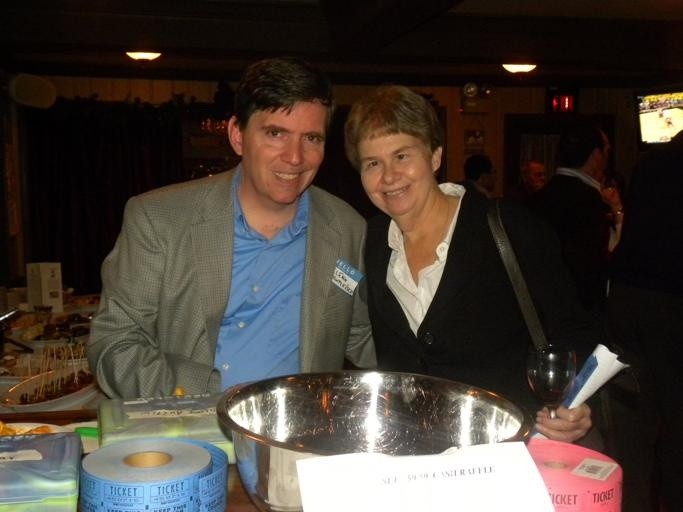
[0,305,99,414]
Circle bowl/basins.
[215,370,533,512]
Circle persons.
[88,59,378,398]
[520,125,624,255]
[344,85,594,442]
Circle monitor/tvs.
[633,88,683,148]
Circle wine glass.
[525,344,577,419]
